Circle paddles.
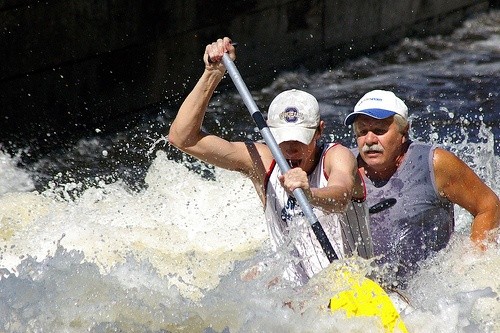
[207,41,409,333]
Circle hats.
[344,90,409,126]
[266,89,320,145]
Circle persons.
[345,90,500,290]
[168,37,376,284]
